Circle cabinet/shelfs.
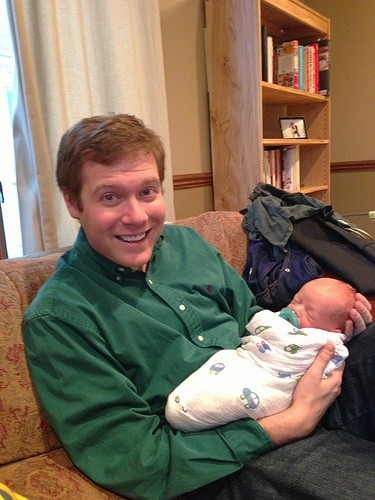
[205,0,331,212]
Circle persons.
[165,279,359,432]
[21,113,375,500]
[289,122,301,137]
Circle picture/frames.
[279,118,307,139]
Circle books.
[259,24,331,98]
[264,145,301,192]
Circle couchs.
[0,181,375,500]
